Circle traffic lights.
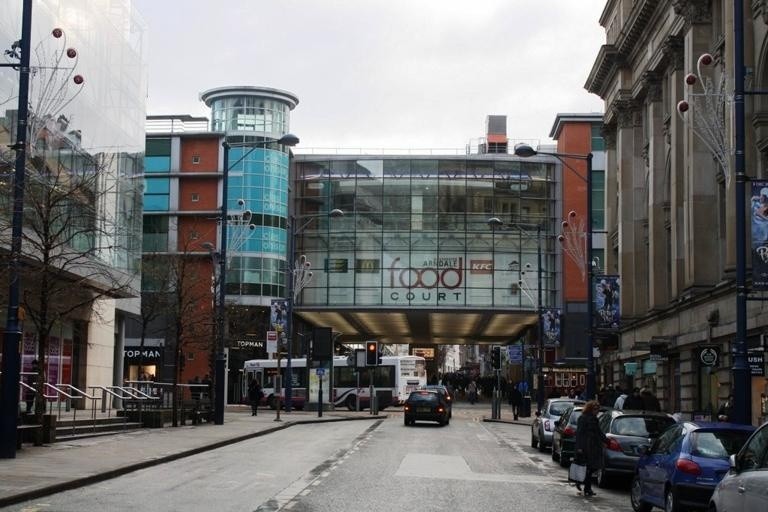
[378,345,383,364]
[368,343,375,362]
[490,346,501,371]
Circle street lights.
[279,207,343,413]
[210,132,300,423]
[488,215,548,414]
[515,142,599,404]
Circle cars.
[402,383,452,427]
[526,389,768,511]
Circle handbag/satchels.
[567,457,589,485]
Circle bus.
[241,350,428,413]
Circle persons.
[752,188,768,221]
[547,386,561,398]
[575,401,609,496]
[202,374,212,409]
[500,377,507,401]
[717,395,736,423]
[26,360,39,412]
[275,305,282,325]
[600,382,661,412]
[247,380,260,416]
[549,314,555,333]
[507,379,516,391]
[432,373,479,404]
[603,284,613,309]
[569,388,579,399]
[189,377,202,412]
[509,386,522,420]
